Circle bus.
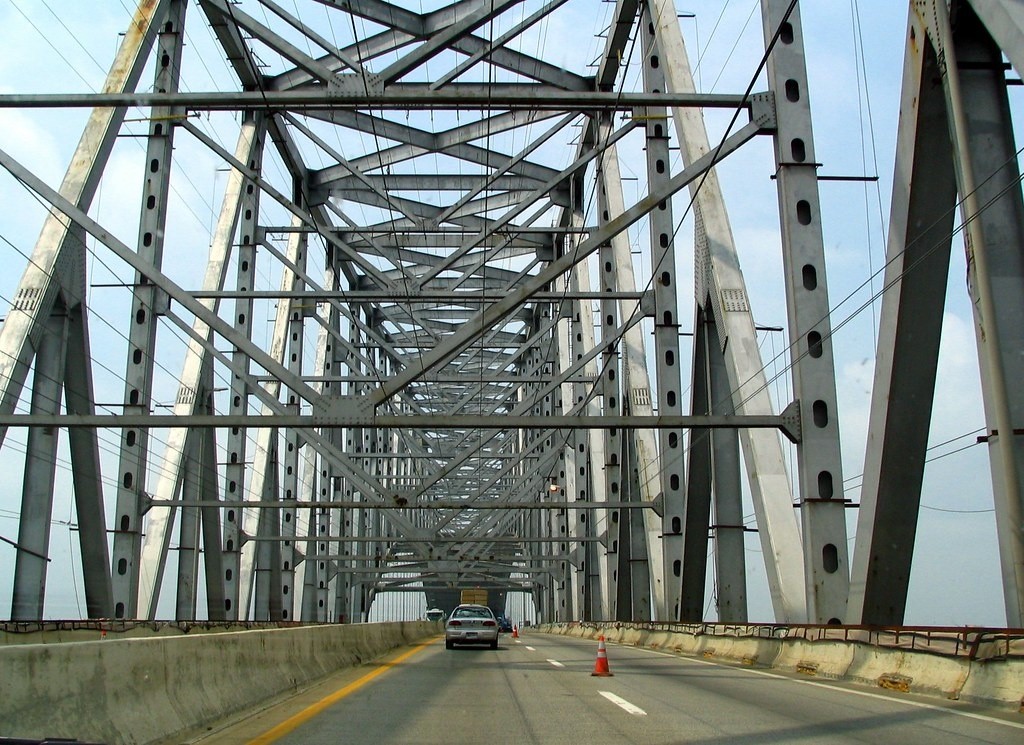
[424,609,445,623]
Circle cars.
[498,619,513,633]
[445,603,500,650]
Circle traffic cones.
[590,634,615,677]
[510,624,519,638]
